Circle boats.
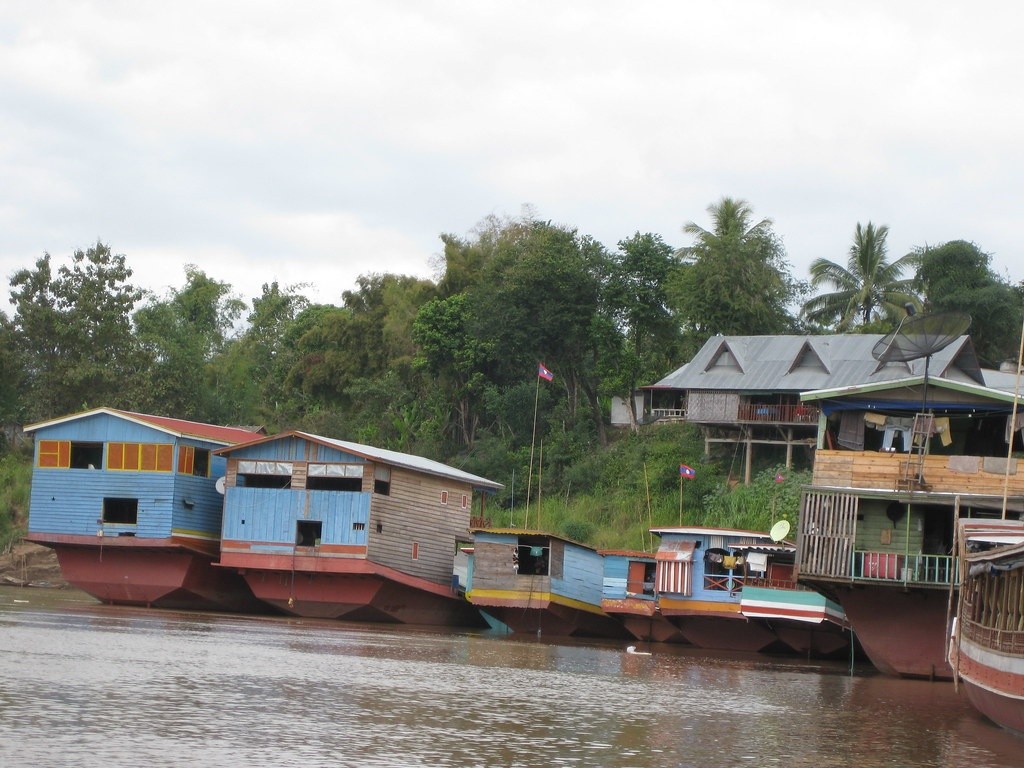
[23,376,1023,743]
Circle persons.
[931,534,991,613]
[756,402,810,424]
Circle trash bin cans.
[901,566,913,581]
[865,552,905,580]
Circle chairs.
[893,462,914,493]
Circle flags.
[539,365,553,381]
[777,474,784,484]
[681,464,695,478]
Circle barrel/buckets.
[900,568,913,581]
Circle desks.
[864,553,903,579]
